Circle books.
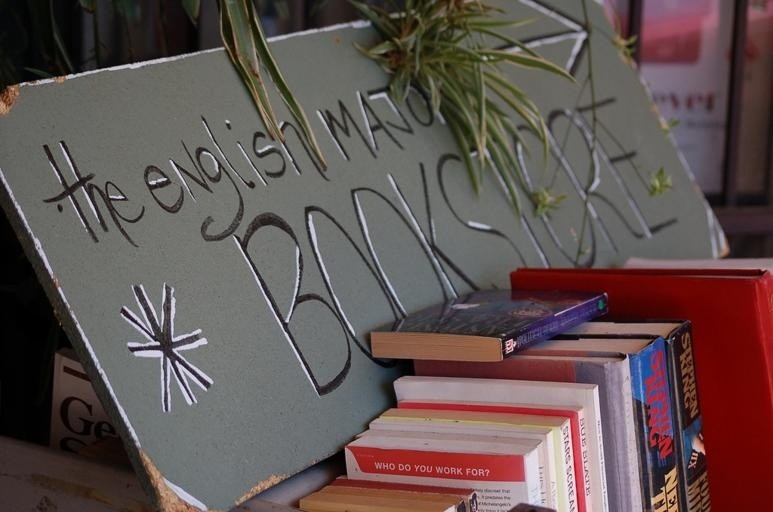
[317,485,467,512]
[48,349,134,470]
[329,478,478,512]
[553,318,711,511]
[300,492,456,512]
[399,398,591,512]
[509,267,773,511]
[380,409,580,511]
[414,349,643,511]
[346,436,540,510]
[373,290,608,361]
[394,377,612,512]
[369,416,557,512]
[356,430,546,510]
[519,333,681,512]
